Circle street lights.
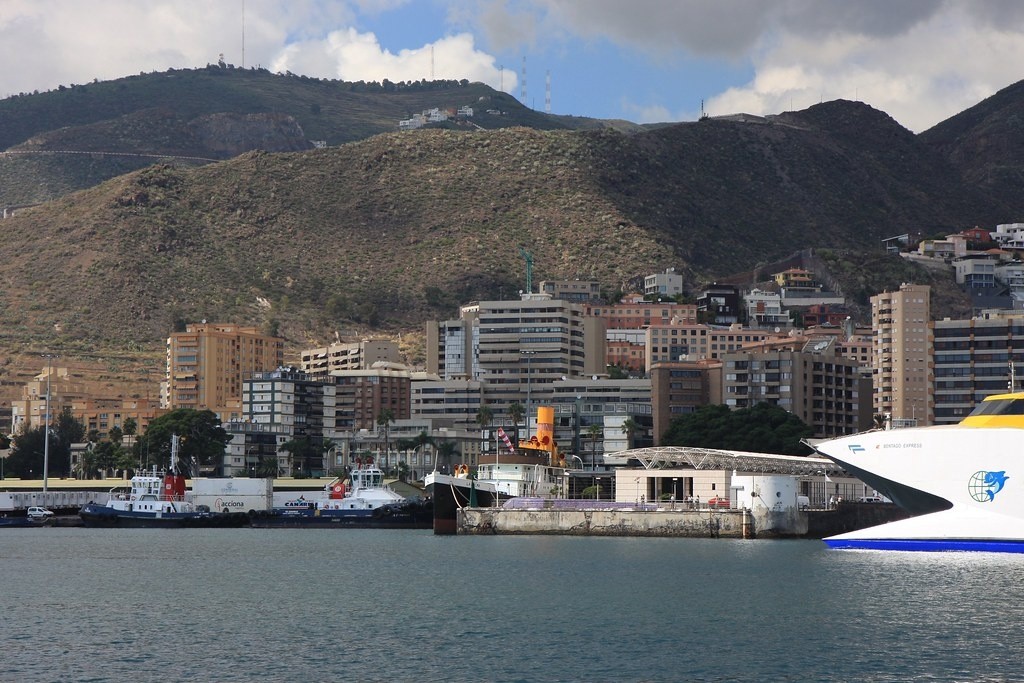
[572,455,584,471]
[410,444,422,482]
[326,444,337,475]
[594,476,602,501]
[522,349,539,440]
[672,477,678,508]
[534,463,539,496]
[39,351,61,493]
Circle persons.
[715,496,719,509]
[670,493,675,509]
[684,494,701,509]
[830,494,842,509]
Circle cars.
[708,497,730,509]
[859,496,881,503]
[27,506,56,517]
[798,496,810,510]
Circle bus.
[347,469,386,478]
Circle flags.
[498,428,514,453]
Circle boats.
[425,407,569,535]
[254,464,434,527]
[799,360,1024,553]
[75,434,251,530]
